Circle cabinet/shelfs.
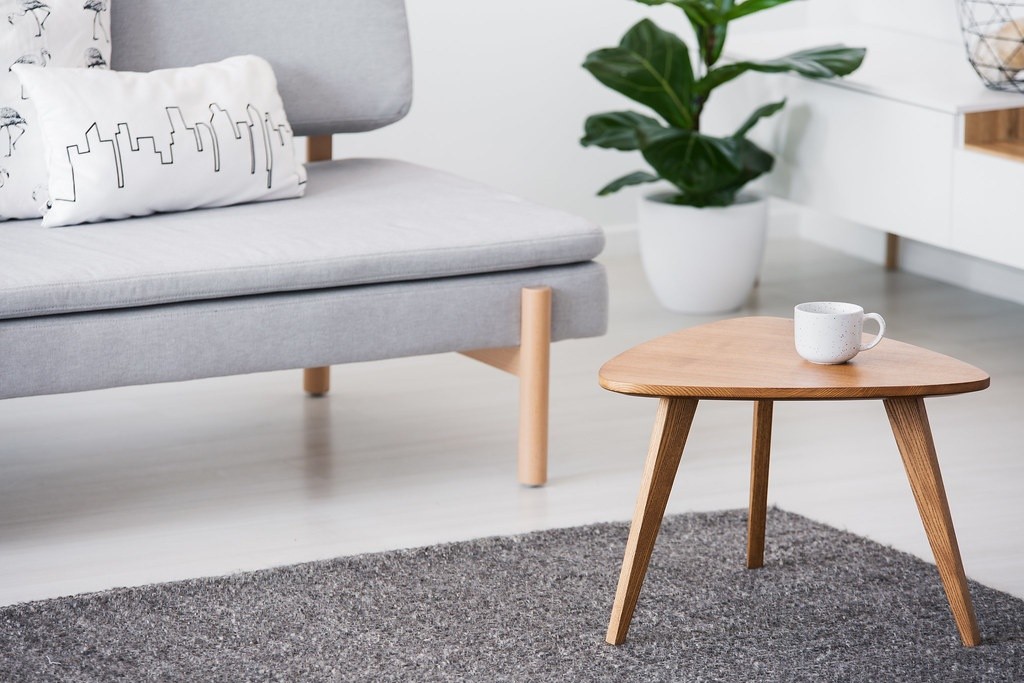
[763,22,1024,308]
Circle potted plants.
[578,0,848,313]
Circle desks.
[598,314,993,647]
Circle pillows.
[9,53,309,231]
[0,0,113,224]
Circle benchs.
[0,0,609,485]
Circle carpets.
[0,504,1024,683]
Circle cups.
[794,301,887,364]
